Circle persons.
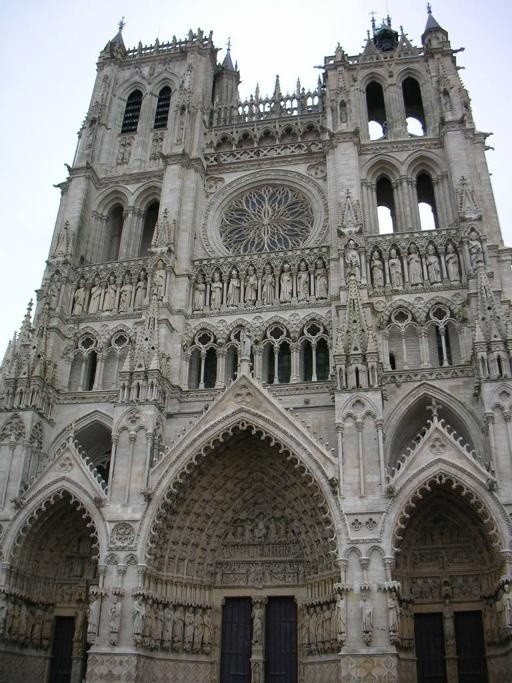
[359,593,374,633]
[302,593,345,648]
[72,595,99,640]
[33,558,81,601]
[108,598,121,645]
[227,515,294,538]
[0,594,55,646]
[387,504,511,646]
[251,603,264,643]
[46,232,486,317]
[215,564,304,585]
[131,597,214,650]
